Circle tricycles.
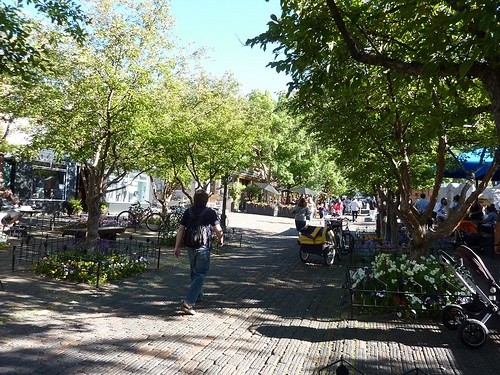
[297,217,354,266]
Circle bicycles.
[116,200,229,231]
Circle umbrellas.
[290,186,317,199]
[254,182,279,196]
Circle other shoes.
[181,302,196,315]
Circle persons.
[369,196,377,222]
[291,195,362,238]
[173,189,224,315]
[415,193,497,250]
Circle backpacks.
[183,208,210,248]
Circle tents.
[421,147,500,197]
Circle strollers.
[438,245,500,349]
